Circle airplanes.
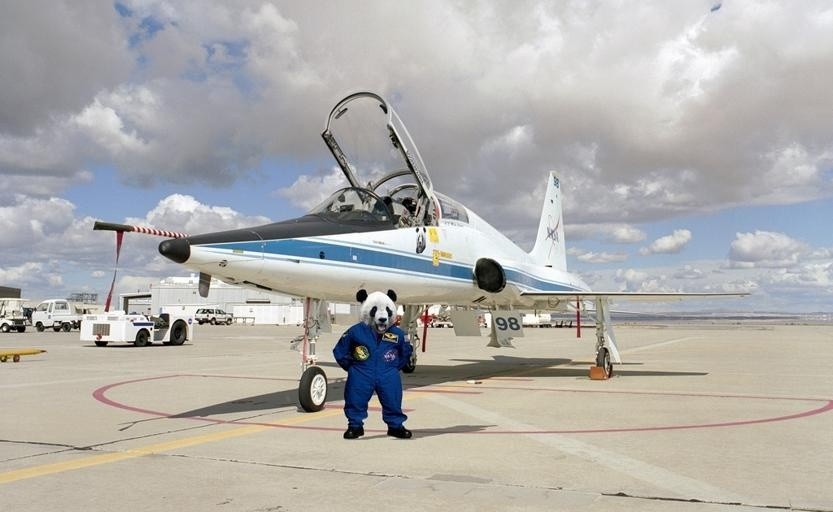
[160,89,751,412]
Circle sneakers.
[344,426,364,439]
[387,426,412,438]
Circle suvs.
[194,308,233,324]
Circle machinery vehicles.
[0,297,193,348]
[427,314,455,328]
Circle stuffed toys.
[333,289,412,439]
